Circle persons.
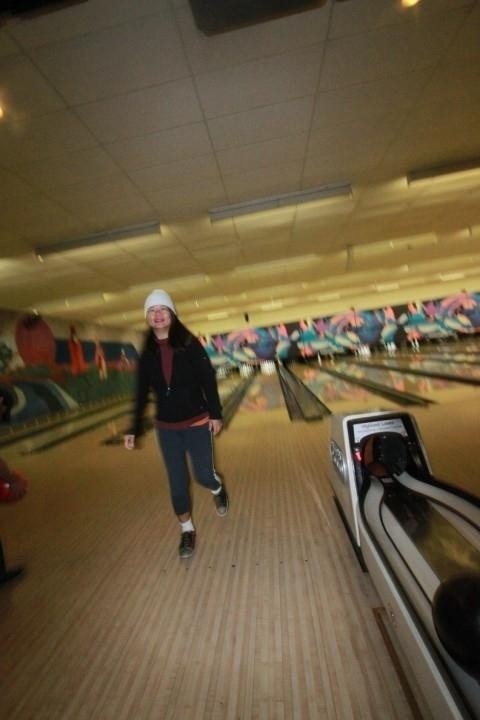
[125,289,228,559]
[0,458,28,590]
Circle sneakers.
[211,484,228,517]
[179,530,195,558]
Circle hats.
[145,289,177,320]
